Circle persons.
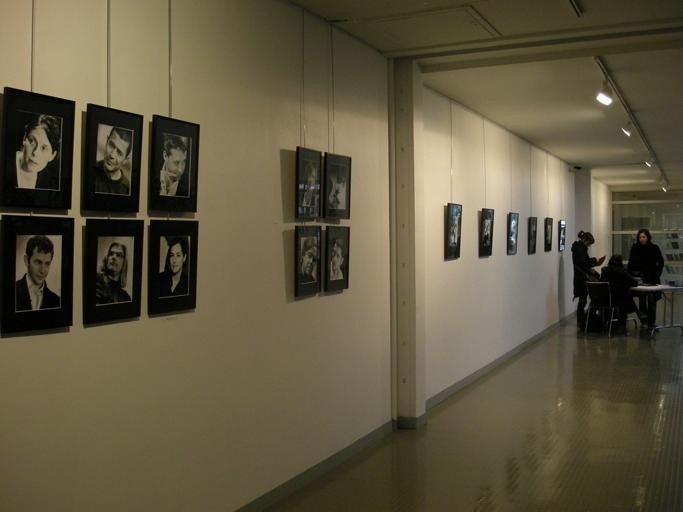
[96,123,133,196]
[546,222,551,245]
[16,236,62,312]
[299,240,318,286]
[626,228,665,330]
[451,207,461,244]
[159,234,194,297]
[143,134,191,198]
[482,212,491,247]
[95,242,131,304]
[558,229,565,245]
[510,215,517,246]
[599,254,648,333]
[571,230,606,328]
[326,174,335,209]
[301,161,319,219]
[530,220,536,240]
[328,239,345,282]
[7,112,63,191]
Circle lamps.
[590,54,672,196]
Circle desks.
[623,280,682,339]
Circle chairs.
[580,280,638,339]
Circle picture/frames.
[0,212,75,335]
[0,82,78,213]
[321,225,351,293]
[80,98,144,217]
[142,216,201,318]
[438,200,567,262]
[145,110,204,215]
[321,151,353,223]
[75,211,144,328]
[289,223,323,299]
[291,141,321,221]
